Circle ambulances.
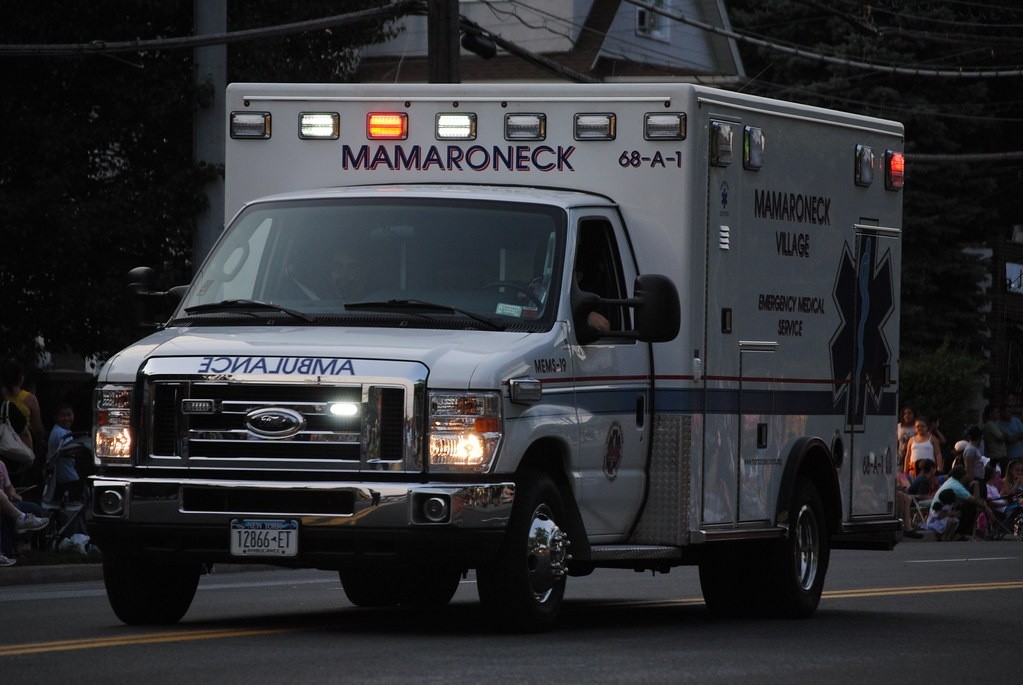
[86,82,908,629]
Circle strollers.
[34,431,100,562]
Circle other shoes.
[904,528,924,539]
[953,535,970,541]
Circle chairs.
[983,493,1023,542]
[897,472,931,527]
[270,227,371,311]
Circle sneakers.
[15,513,49,534]
[0,554,17,566]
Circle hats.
[954,439,969,452]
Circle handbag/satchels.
[0,400,35,464]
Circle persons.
[897,404,1023,542]
[47,403,79,502]
[515,251,611,333]
[0,363,50,567]
